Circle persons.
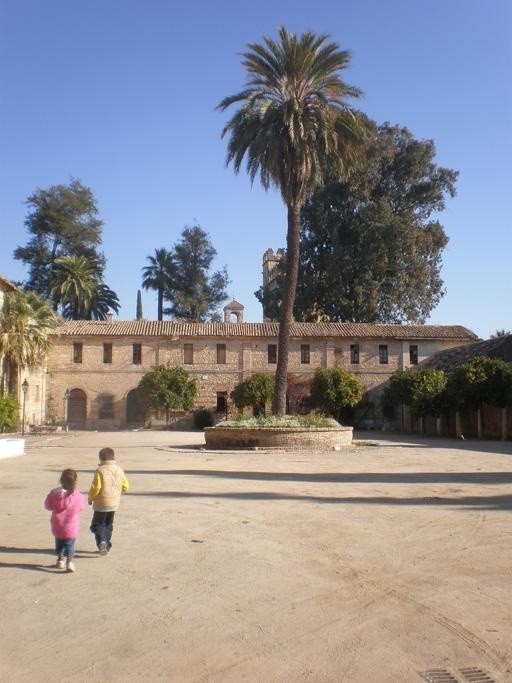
[87,447,129,555]
[44,469,85,572]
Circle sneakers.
[66,562,74,572]
[56,558,65,569]
[99,542,109,557]
